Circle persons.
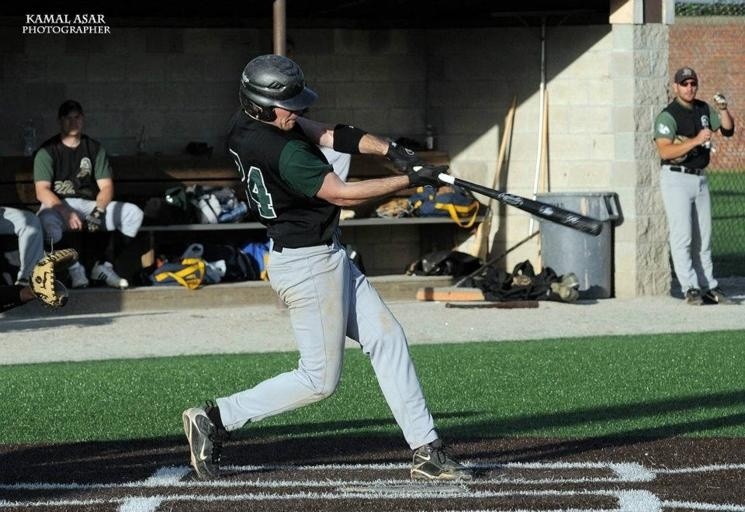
[651,66,734,305]
[0,101,143,312]
[182,55,477,482]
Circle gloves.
[83,207,105,232]
[385,142,427,175]
[408,163,449,189]
[713,91,727,109]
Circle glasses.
[680,81,697,86]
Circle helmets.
[239,54,320,121]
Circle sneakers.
[181,400,230,479]
[687,288,704,305]
[410,439,471,481]
[90,260,129,288]
[68,261,89,289]
[706,288,731,305]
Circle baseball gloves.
[377,198,412,217]
[31,249,78,309]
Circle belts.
[273,228,342,252]
[670,167,700,175]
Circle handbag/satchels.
[415,249,481,275]
[410,183,479,216]
[149,242,269,290]
[144,197,198,223]
[474,262,579,302]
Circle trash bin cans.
[534,192,622,299]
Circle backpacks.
[186,184,249,223]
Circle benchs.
[0,149,451,271]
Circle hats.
[58,100,82,118]
[675,68,697,83]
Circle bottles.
[423,124,445,150]
[22,119,37,155]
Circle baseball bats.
[414,166,601,237]
[445,301,539,308]
